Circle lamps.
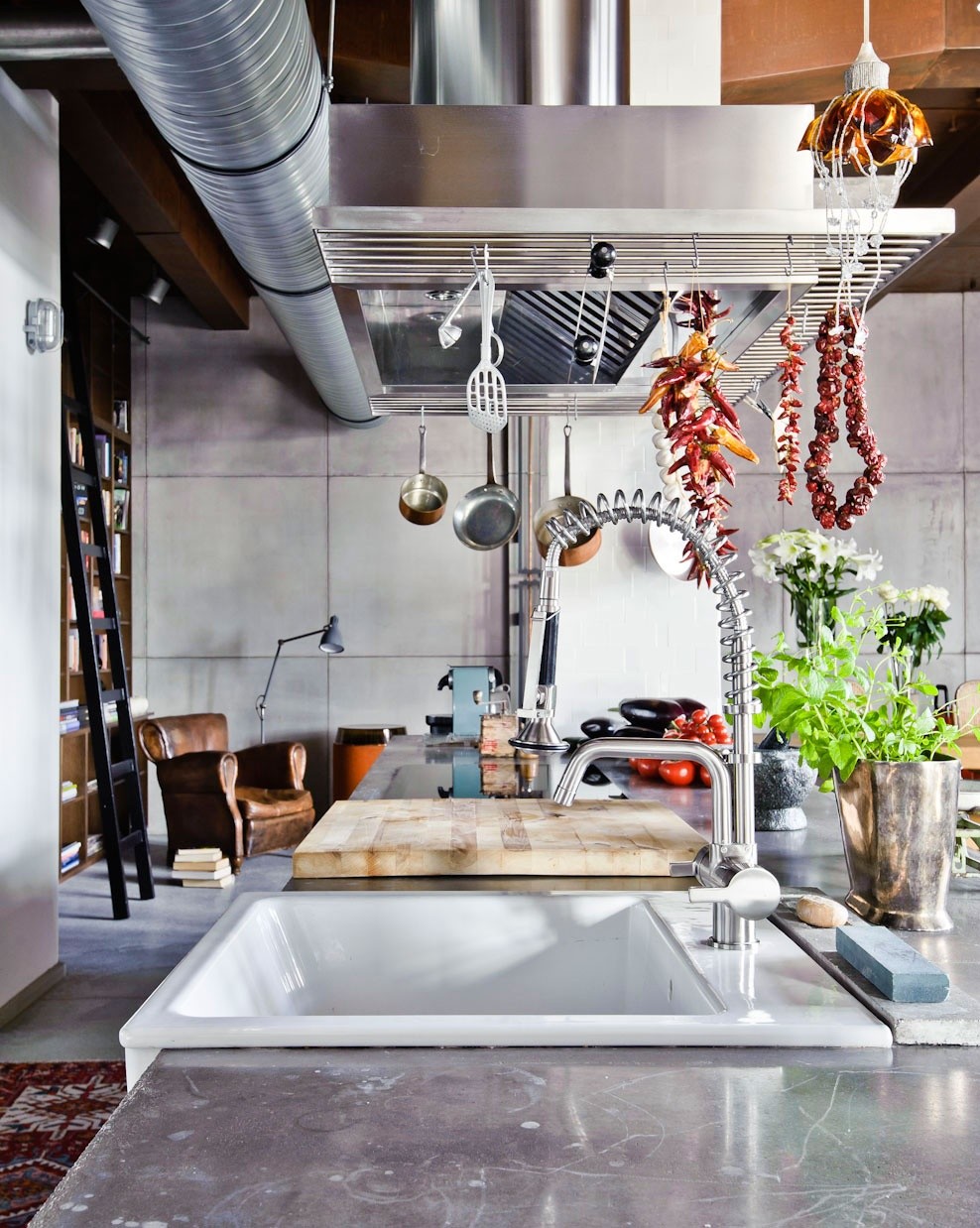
[25,297,61,353]
[139,275,170,308]
[255,615,344,743]
[84,217,120,252]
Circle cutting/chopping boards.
[291,796,710,879]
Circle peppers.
[777,305,887,530]
[639,290,759,589]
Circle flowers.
[745,523,883,651]
[864,579,953,690]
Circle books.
[60,699,118,735]
[171,847,235,889]
[61,841,82,874]
[62,780,77,801]
[87,834,102,856]
[65,399,130,672]
[87,779,98,794]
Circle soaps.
[794,891,850,928]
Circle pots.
[399,425,448,526]
[532,424,601,568]
[453,431,522,550]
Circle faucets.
[504,486,786,951]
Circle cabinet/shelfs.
[57,261,149,882]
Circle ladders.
[61,331,154,920]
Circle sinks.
[117,884,896,1094]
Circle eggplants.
[562,697,709,786]
[758,726,791,750]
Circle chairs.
[139,713,316,877]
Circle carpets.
[0,1059,126,1228]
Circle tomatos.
[629,709,735,786]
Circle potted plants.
[724,588,980,934]
